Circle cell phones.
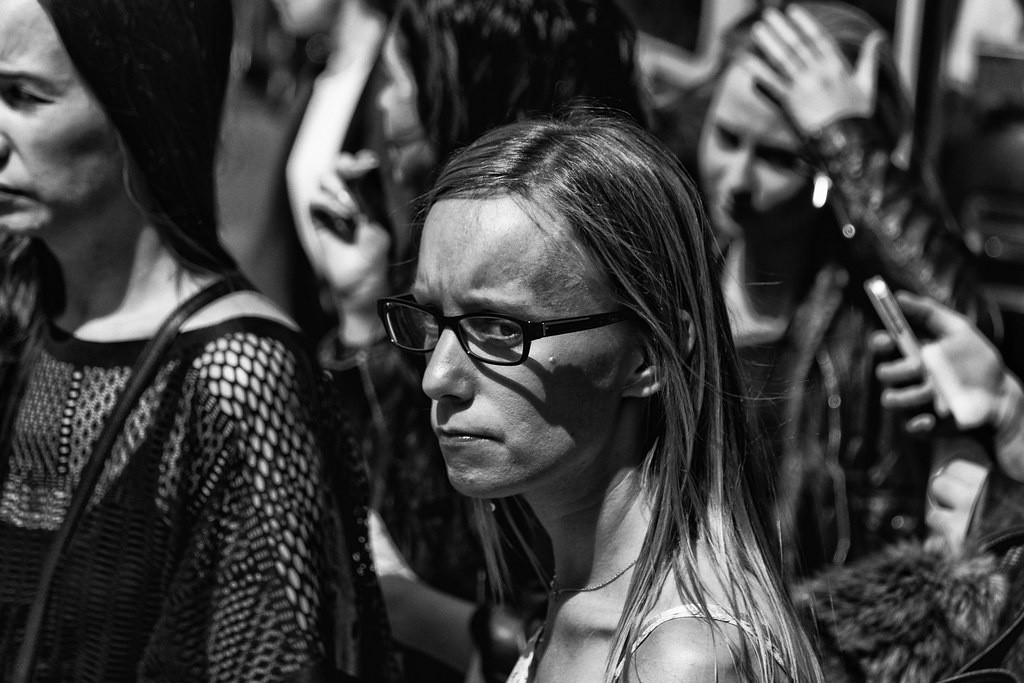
[865,275,951,422]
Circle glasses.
[379,294,632,366]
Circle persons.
[0,0,1024,683]
[376,114,823,683]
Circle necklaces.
[550,560,637,600]
